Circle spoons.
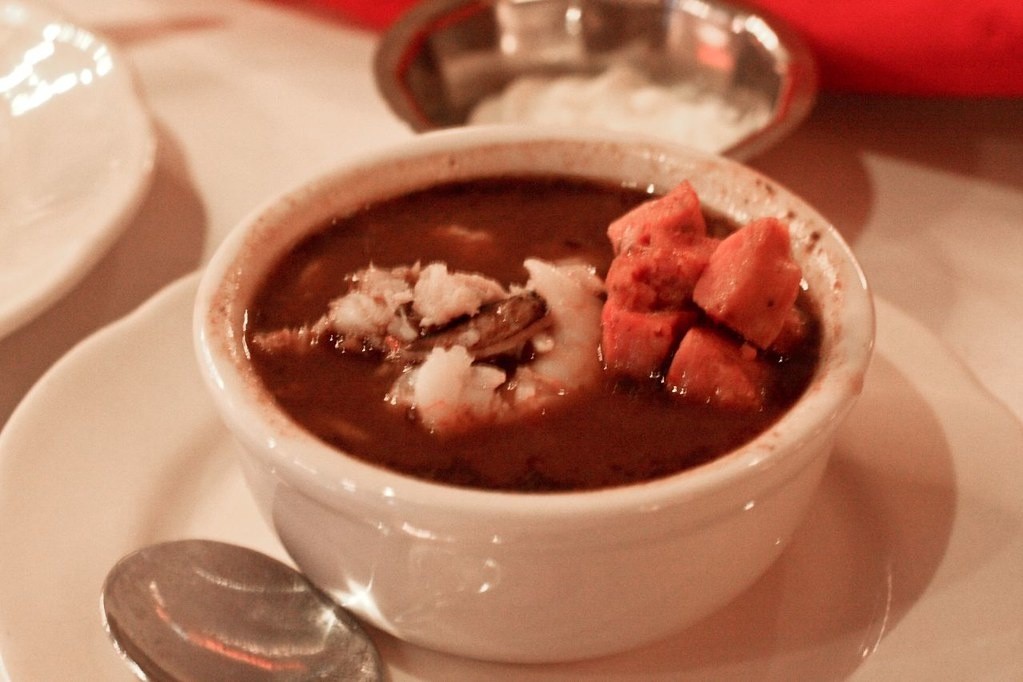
[103,540,385,682]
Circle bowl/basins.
[371,0,818,163]
[192,122,877,664]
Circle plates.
[0,266,1022,682]
[0,2,157,337]
